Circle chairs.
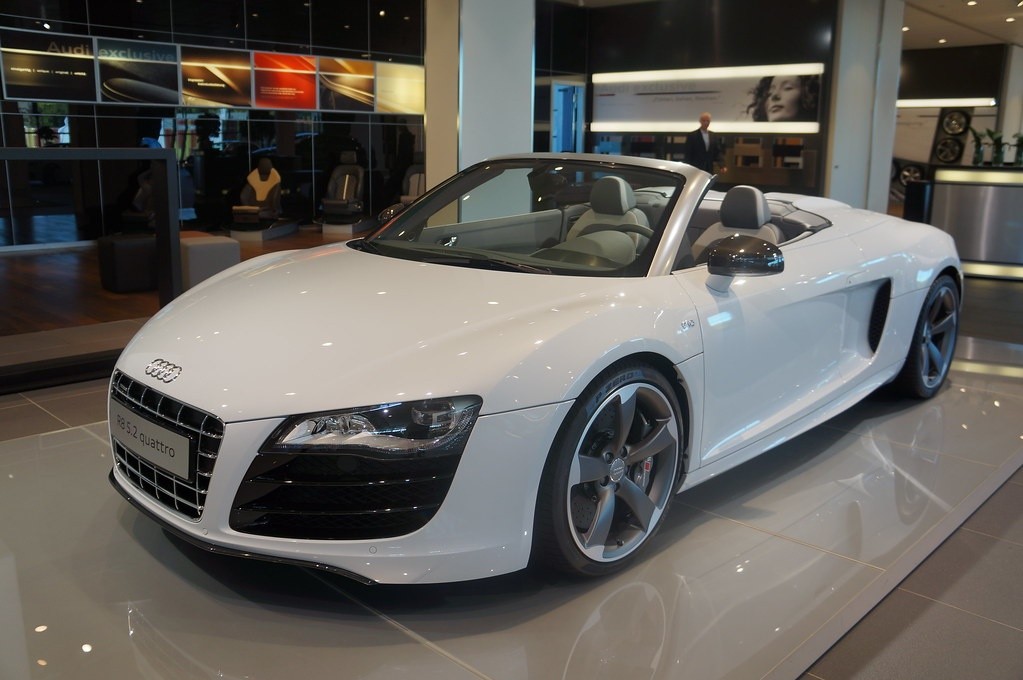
[321,148,369,223]
[558,175,786,264]
[399,160,426,205]
[230,158,284,226]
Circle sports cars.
[105,150,969,604]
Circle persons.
[744,76,820,122]
[684,113,728,191]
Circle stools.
[100,228,242,297]
